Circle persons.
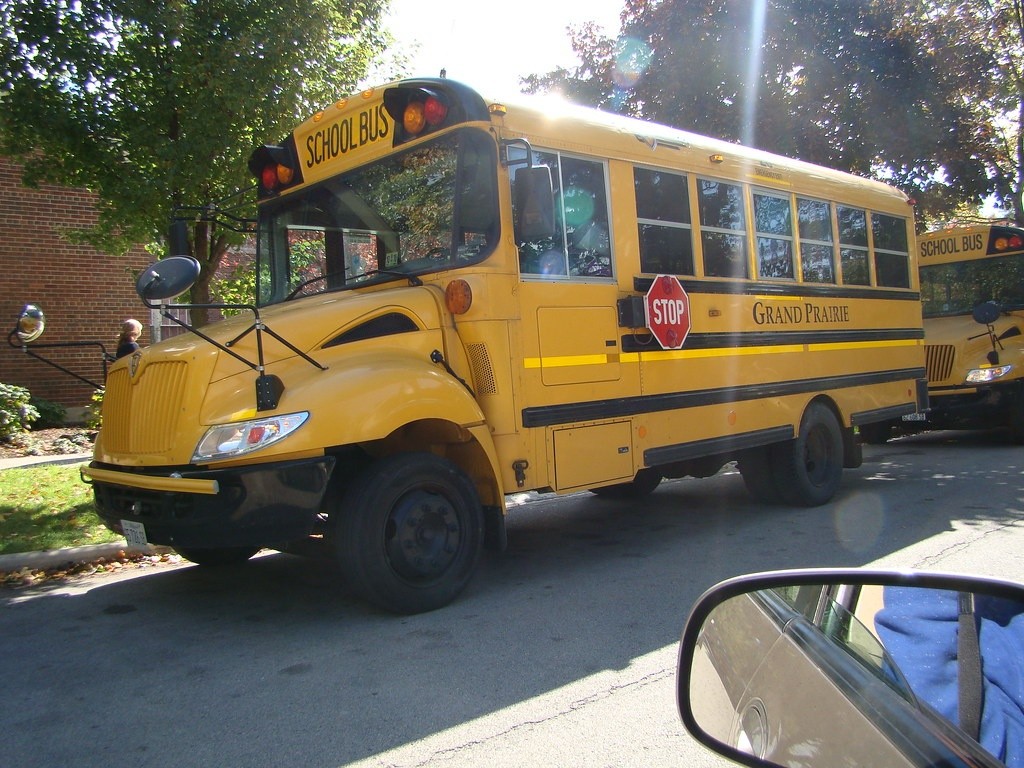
[116,319,143,358]
[874,585,1024,768]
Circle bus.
[0,67,928,615]
[857,222,1024,447]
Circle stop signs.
[641,274,692,351]
